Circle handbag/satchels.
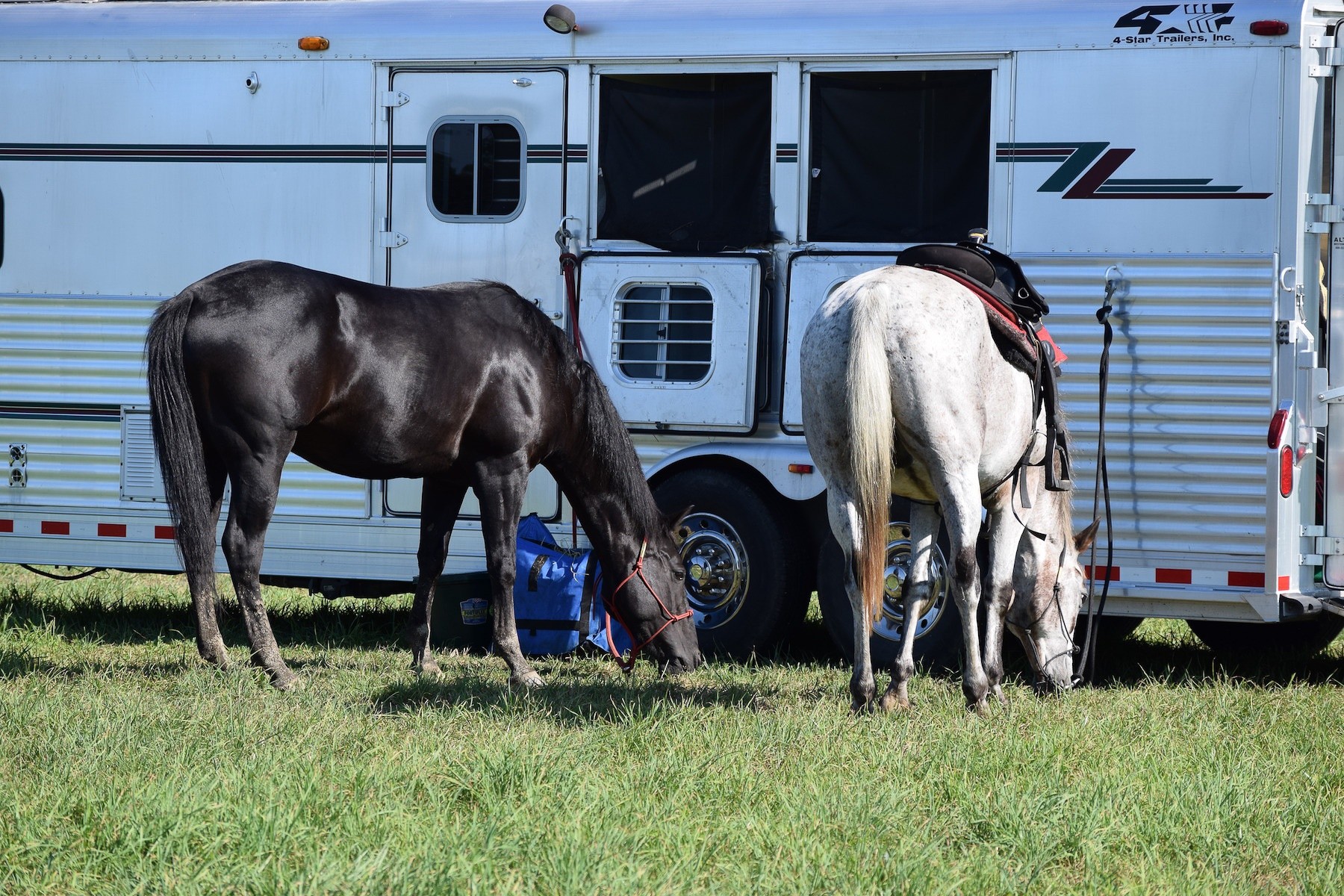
[512,513,635,661]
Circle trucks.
[0,0,1343,678]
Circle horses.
[799,240,1103,715]
[148,261,700,697]
